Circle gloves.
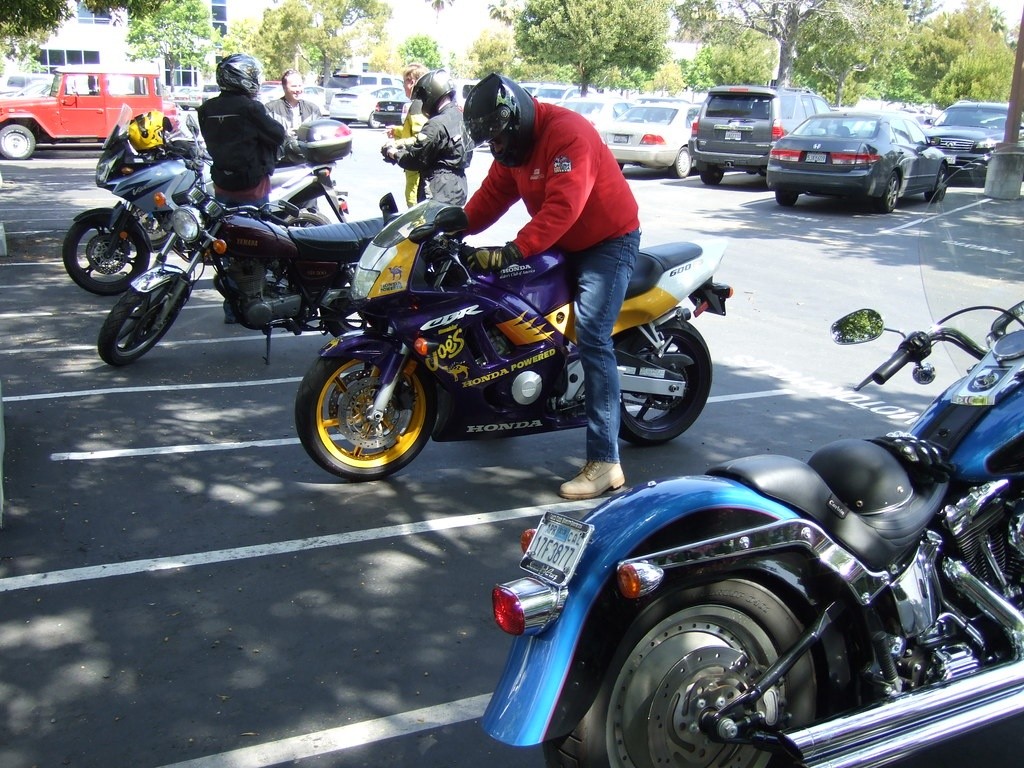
[381,144,400,165]
[466,241,524,275]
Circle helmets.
[410,69,456,119]
[463,72,535,168]
[216,53,264,97]
[128,109,164,153]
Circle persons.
[197,53,285,323]
[381,69,474,207]
[265,69,322,228]
[444,73,640,500]
[386,62,429,208]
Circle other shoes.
[224,315,236,323]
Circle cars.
[0,69,485,131]
[766,109,950,214]
[518,78,945,179]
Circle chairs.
[835,127,850,137]
[384,91,390,98]
[752,102,769,115]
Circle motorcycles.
[294,192,732,483]
[62,101,352,296]
[97,149,412,368]
[479,293,1024,768]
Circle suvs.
[922,100,1024,189]
[0,63,178,162]
[688,84,833,186]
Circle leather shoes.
[559,459,625,500]
